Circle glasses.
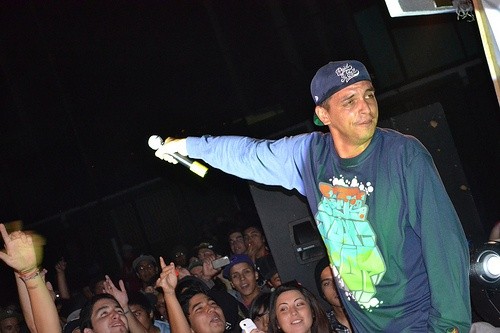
[175,252,184,258]
[136,263,154,270]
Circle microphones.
[148,135,209,178]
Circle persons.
[1,221,356,333]
[469,221,500,333]
[155,62,473,333]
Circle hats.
[132,253,157,270]
[223,253,254,278]
[310,60,371,126]
[193,239,216,252]
[188,262,203,271]
[0,302,23,320]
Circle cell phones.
[212,255,230,268]
[239,318,257,333]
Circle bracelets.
[19,266,39,281]
[125,311,129,314]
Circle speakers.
[246,182,334,314]
